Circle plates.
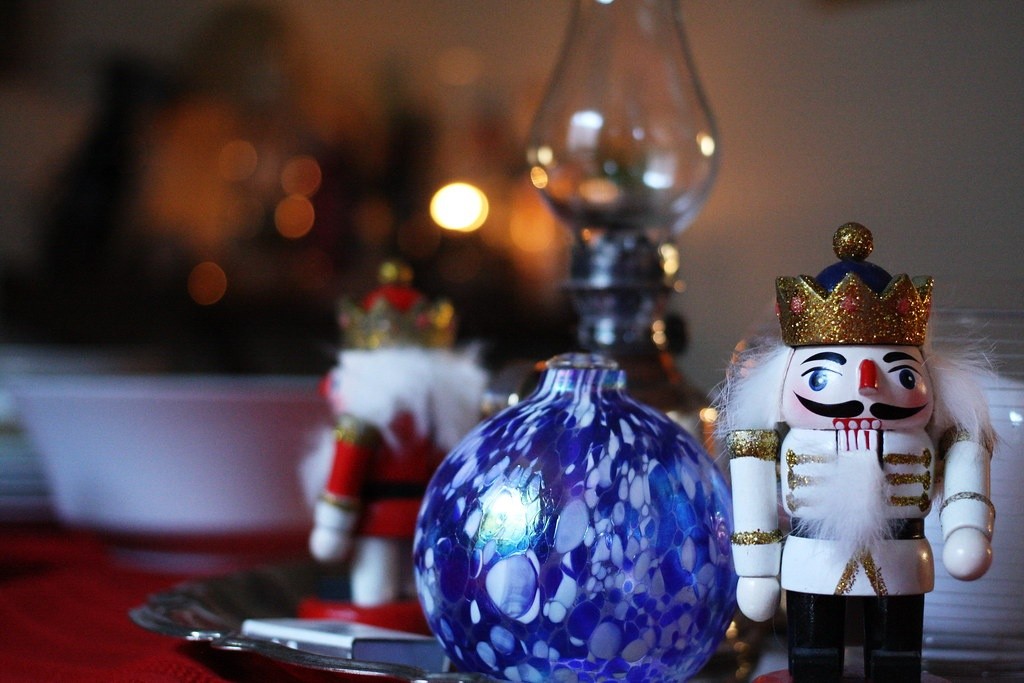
[129,570,472,683]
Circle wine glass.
[520,0,721,415]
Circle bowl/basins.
[22,376,336,569]
